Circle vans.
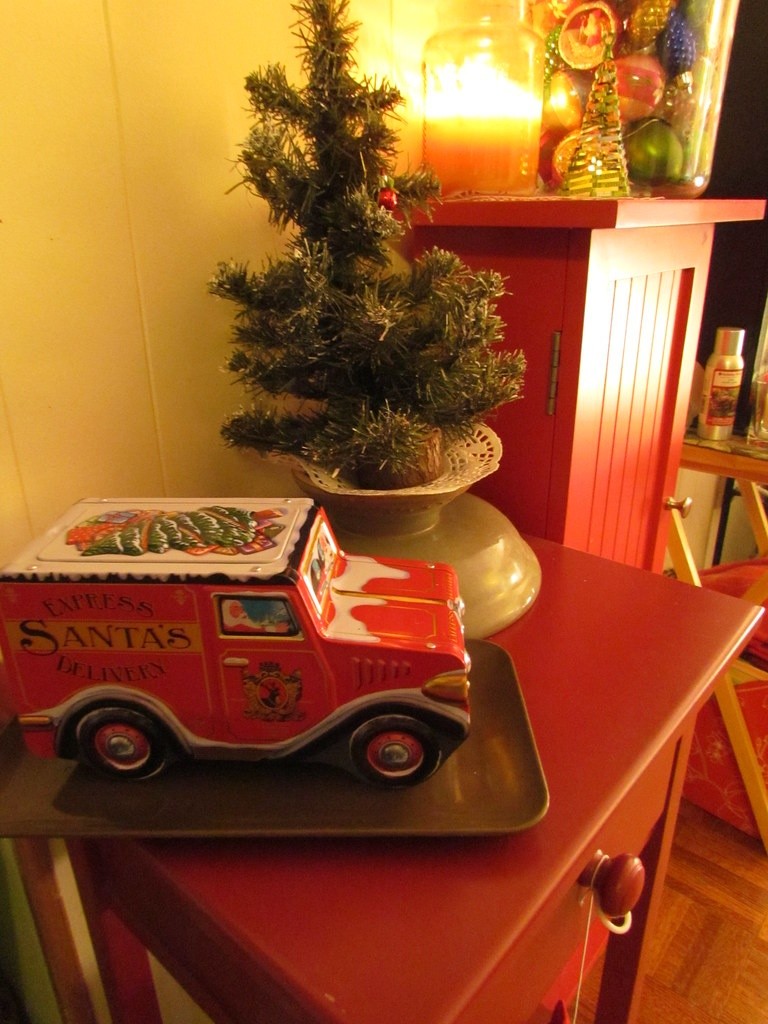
[0,497,472,787]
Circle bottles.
[746,295,768,449]
[698,327,745,441]
[422,1,545,196]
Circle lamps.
[419,17,548,196]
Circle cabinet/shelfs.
[383,194,765,578]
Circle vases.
[284,467,544,644]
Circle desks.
[63,537,768,1024]
[667,424,768,558]
